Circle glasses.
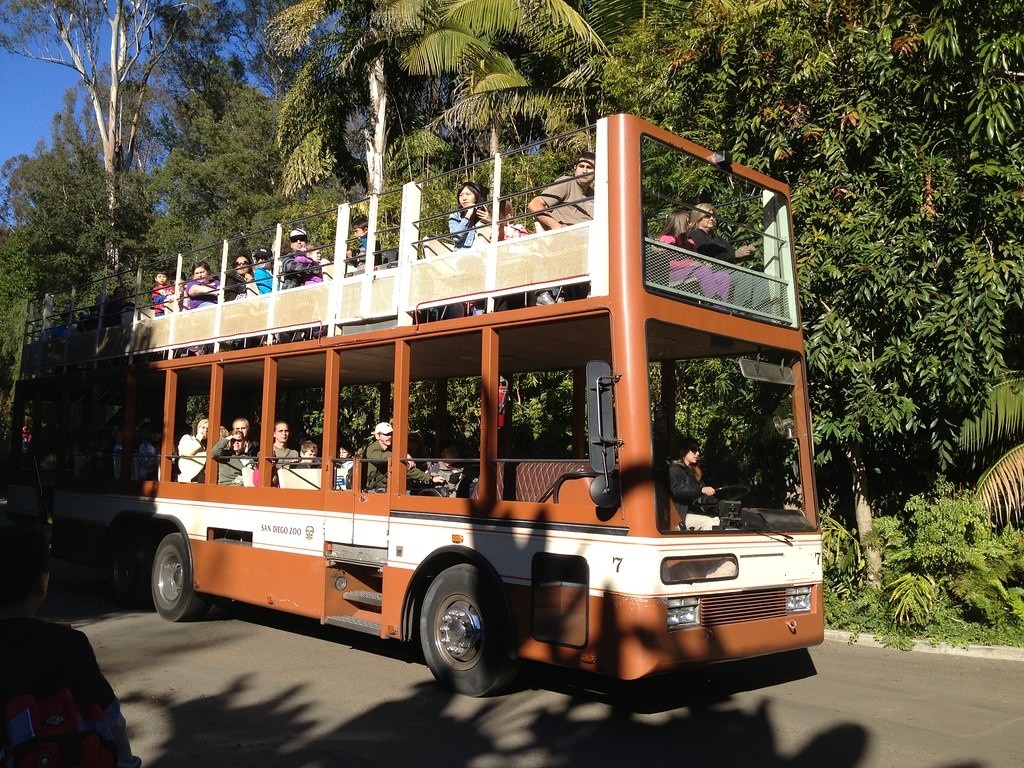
[687,447,700,454]
[293,237,306,242]
[256,256,269,261]
[234,262,249,267]
[704,213,715,219]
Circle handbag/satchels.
[120,308,133,324]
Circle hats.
[253,248,271,256]
[288,229,307,238]
[374,422,394,436]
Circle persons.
[253,454,279,487]
[100,422,177,480]
[670,439,720,521]
[282,229,325,340]
[171,418,260,486]
[659,203,768,319]
[60,286,134,371]
[448,182,565,316]
[527,151,595,298]
[0,526,142,768]
[273,421,322,484]
[151,248,282,358]
[346,218,381,275]
[338,422,479,498]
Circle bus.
[7,113,825,697]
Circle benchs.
[119,217,546,325]
[241,461,596,504]
